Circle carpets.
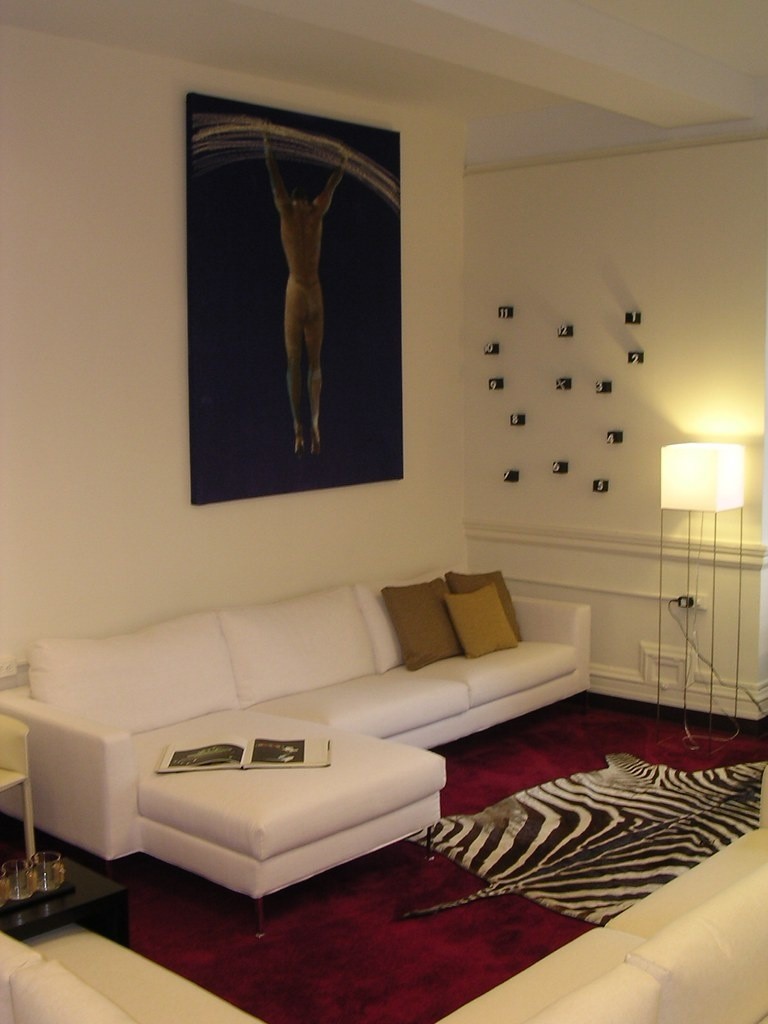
[397,753,768,927]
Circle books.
[155,729,333,774]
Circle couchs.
[0,578,594,936]
[437,765,768,1024]
[0,923,267,1024]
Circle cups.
[0,867,10,908]
[31,851,65,893]
[2,859,34,899]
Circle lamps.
[653,442,745,759]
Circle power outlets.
[685,593,708,609]
[0,656,17,678]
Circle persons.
[261,118,351,456]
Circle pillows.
[444,570,523,642]
[380,577,463,671]
[444,581,518,659]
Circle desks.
[0,715,34,861]
[0,853,130,947]
